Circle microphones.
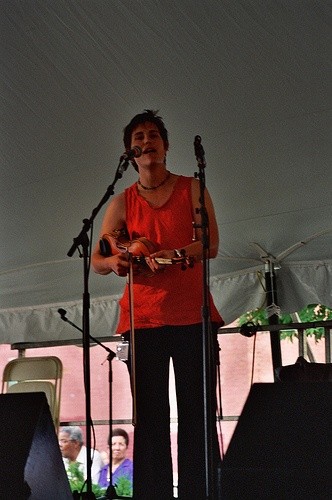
[193,137,206,167]
[123,145,142,160]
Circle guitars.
[101,228,195,276]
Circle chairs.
[1,356,63,437]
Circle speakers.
[221,383,332,500]
[0,392,74,500]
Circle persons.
[92,109,225,499]
[58,426,102,485]
[98,429,133,491]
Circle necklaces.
[138,171,171,190]
